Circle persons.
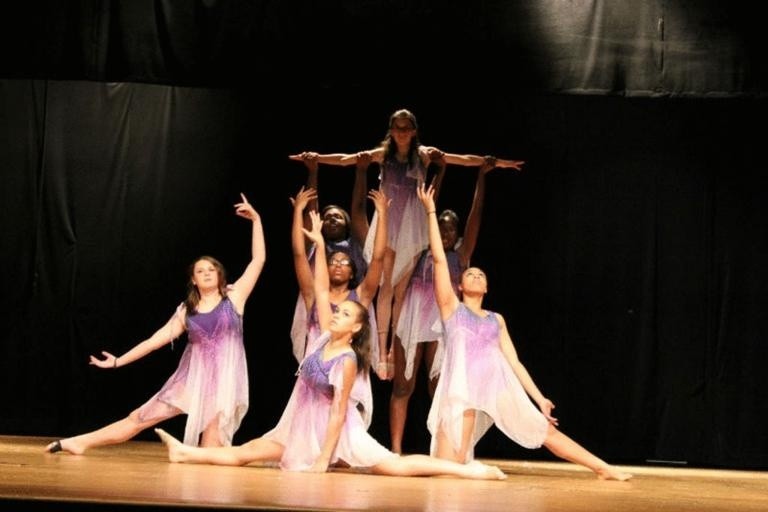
[47,194,266,456]
[417,176,633,482]
[289,109,524,379]
[290,152,497,455]
[153,209,508,479]
